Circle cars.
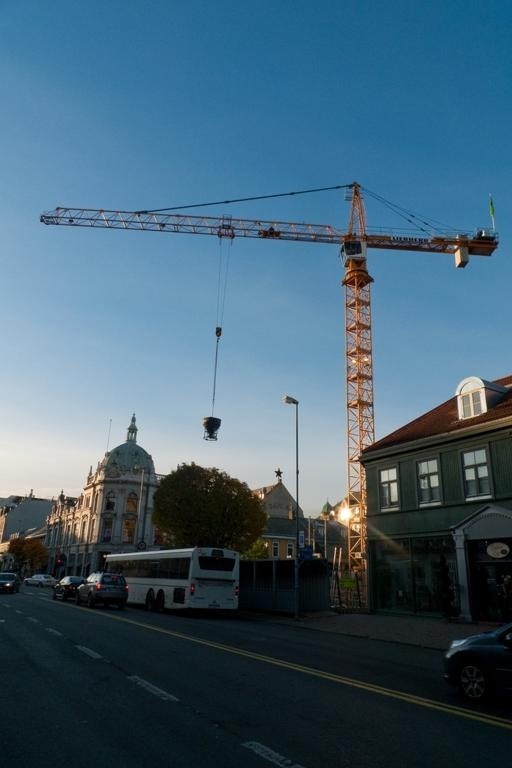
[51,576,85,601]
[0,573,22,594]
[437,622,512,700]
[24,574,57,587]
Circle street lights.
[137,465,151,550]
[283,394,303,621]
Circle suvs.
[75,570,129,609]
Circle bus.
[103,547,241,612]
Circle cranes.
[41,184,500,613]
